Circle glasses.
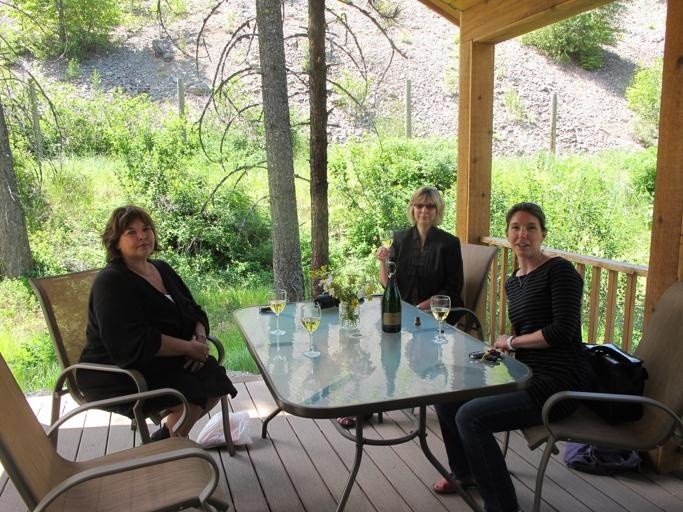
[413,203,436,210]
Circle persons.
[76,204,237,442]
[336,185,466,427]
[432,201,585,512]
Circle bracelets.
[507,334,519,352]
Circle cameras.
[314,292,364,309]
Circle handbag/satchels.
[581,343,645,426]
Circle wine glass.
[429,295,451,344]
[266,288,288,337]
[426,344,448,387]
[300,358,322,406]
[297,301,322,358]
[268,337,288,379]
[379,230,394,264]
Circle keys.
[466,346,504,362]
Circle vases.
[338,302,360,328]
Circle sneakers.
[151,423,171,441]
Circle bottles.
[379,336,400,398]
[380,264,402,334]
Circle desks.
[230,291,533,512]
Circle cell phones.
[469,350,485,358]
[259,306,271,313]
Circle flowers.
[307,261,375,325]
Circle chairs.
[28,264,241,458]
[500,279,682,511]
[401,241,499,343]
[1,357,231,512]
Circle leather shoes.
[337,413,373,428]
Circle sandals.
[432,473,474,494]
